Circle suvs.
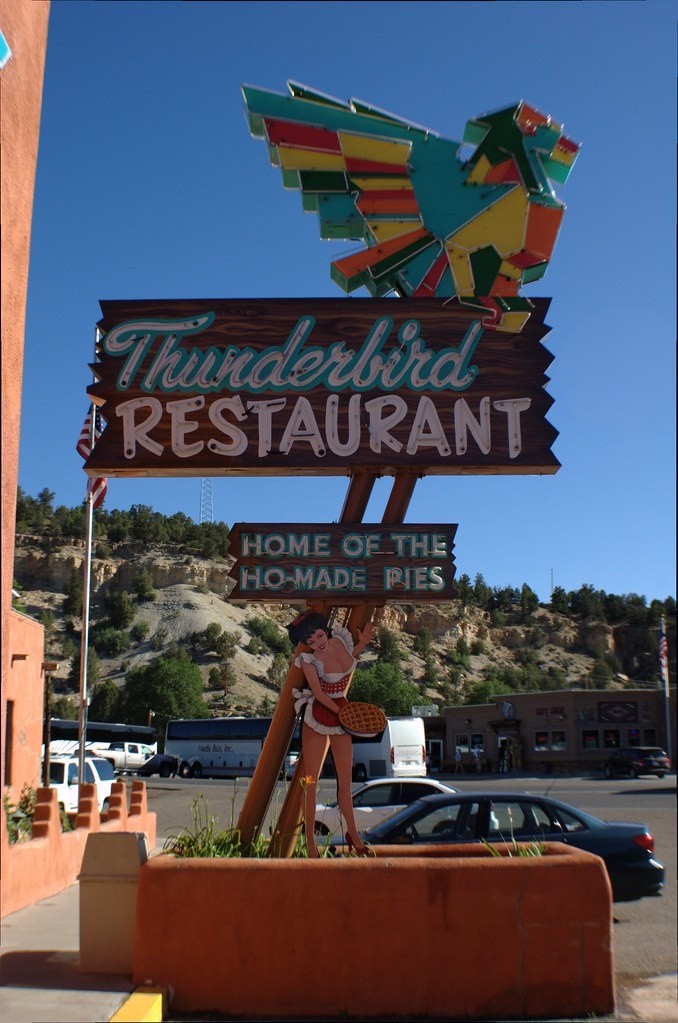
[40,755,117,815]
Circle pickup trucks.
[75,739,179,778]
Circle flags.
[658,631,668,680]
[76,403,107,512]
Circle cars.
[318,790,666,902]
[301,776,500,841]
[605,745,672,779]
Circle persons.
[496,743,512,775]
[454,749,464,775]
[426,753,432,778]
[472,745,482,777]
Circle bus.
[41,716,158,760]
[322,715,428,782]
[164,716,325,782]
